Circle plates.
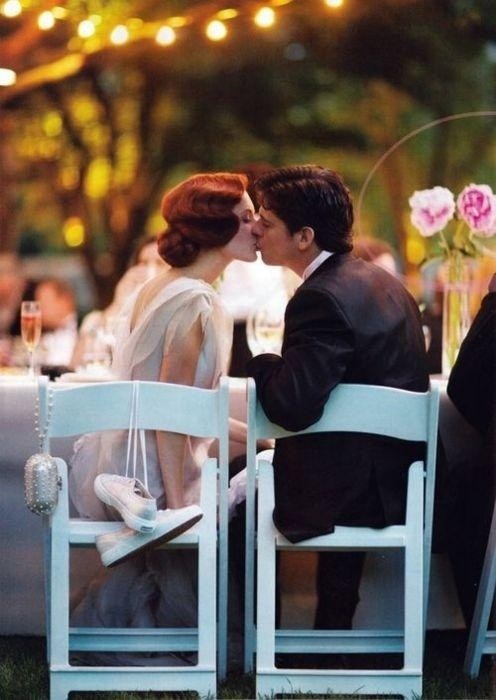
[55,371,114,384]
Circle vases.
[440,288,473,376]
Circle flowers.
[408,180,496,369]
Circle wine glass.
[21,301,42,379]
[247,290,296,358]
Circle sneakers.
[94,473,154,531]
[94,505,202,567]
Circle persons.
[229,164,428,669]
[442,272,496,679]
[67,170,276,670]
[1,158,445,371]
